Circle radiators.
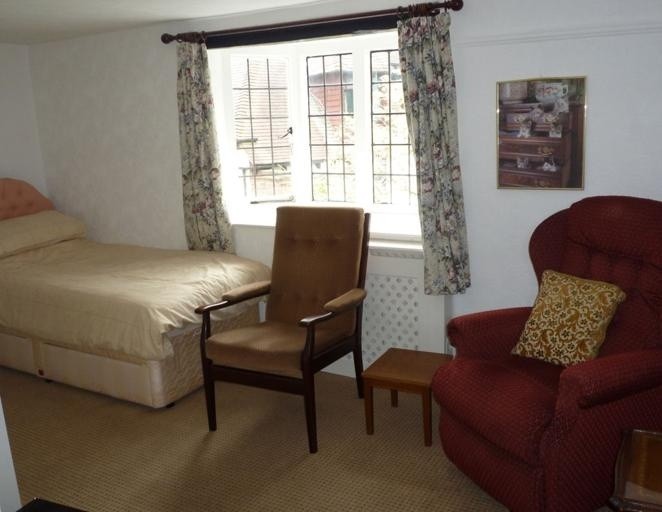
[322,240,446,380]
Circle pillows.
[511,269,626,367]
[1,211,87,258]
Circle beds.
[0,177,272,410]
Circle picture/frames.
[496,77,586,191]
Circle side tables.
[360,347,454,447]
[609,424,662,512]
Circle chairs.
[193,206,371,454]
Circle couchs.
[430,196,661,512]
